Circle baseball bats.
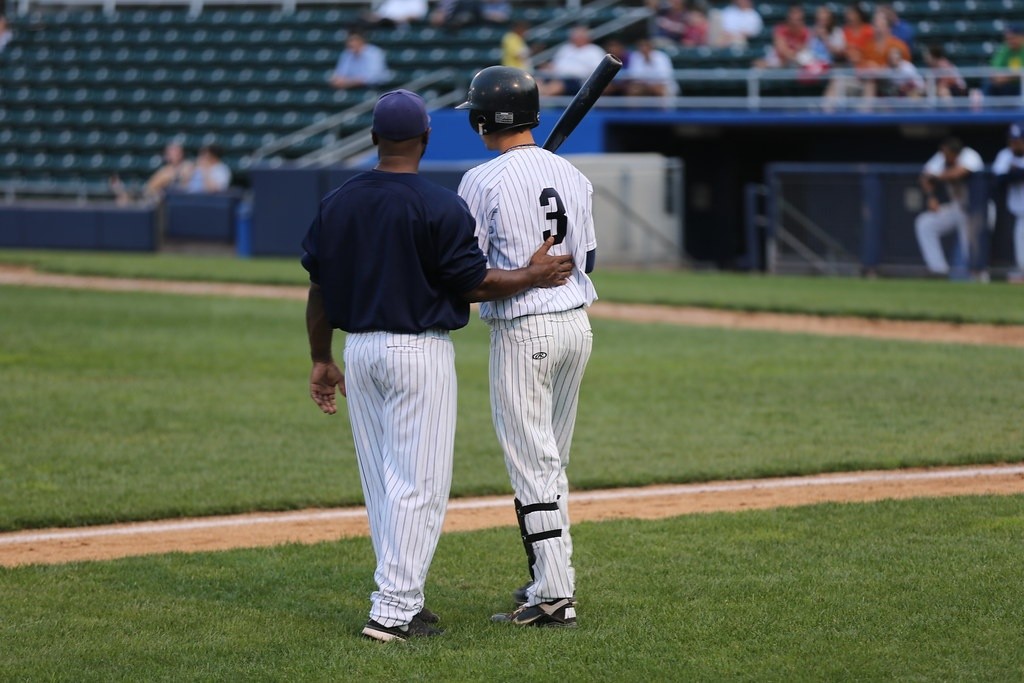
[541,53,625,153]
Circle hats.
[371,88,431,139]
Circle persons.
[299,90,573,644]
[884,45,925,98]
[533,24,607,96]
[502,15,530,76]
[987,122,1024,277]
[969,23,1024,112]
[911,132,999,282]
[604,36,681,97]
[328,30,401,87]
[645,0,765,48]
[353,0,512,30]
[749,3,926,99]
[456,66,599,629]
[917,40,967,98]
[136,133,231,194]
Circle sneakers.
[513,580,578,605]
[361,615,445,643]
[415,607,440,624]
[490,598,578,628]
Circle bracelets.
[925,190,936,198]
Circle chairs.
[0,0,1024,202]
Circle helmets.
[454,66,541,135]
